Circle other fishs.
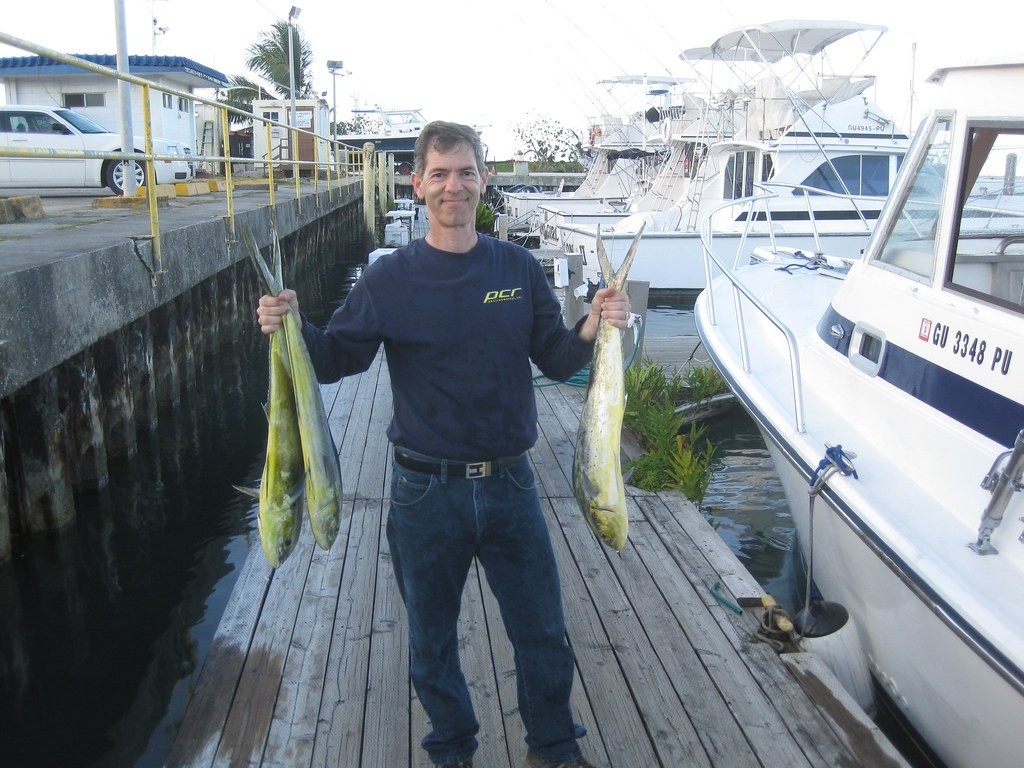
[571,221,647,552]
[240,215,345,568]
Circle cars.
[0,104,197,195]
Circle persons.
[257,121,632,768]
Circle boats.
[486,21,1024,298]
[695,61,1024,768]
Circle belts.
[394,449,526,479]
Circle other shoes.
[526,746,593,768]
[435,758,473,768]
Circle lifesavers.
[589,127,601,144]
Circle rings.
[625,312,628,320]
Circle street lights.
[150,16,169,56]
[287,5,301,177]
[327,59,344,171]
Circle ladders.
[199,120,214,176]
